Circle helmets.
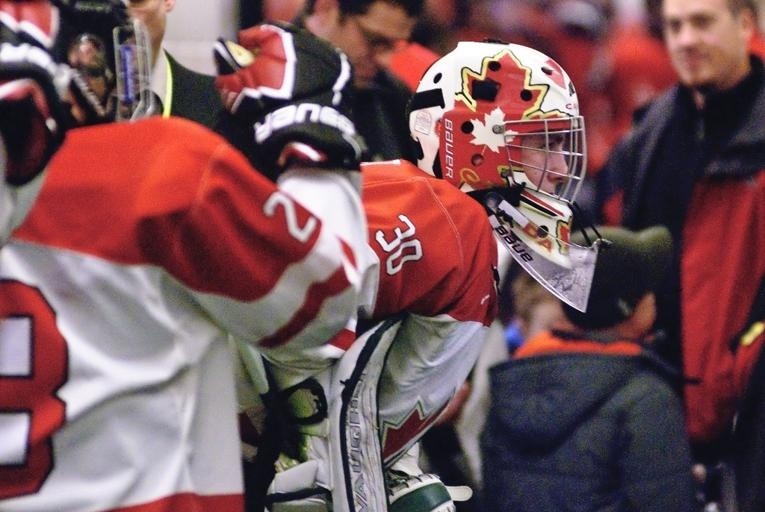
[402,37,589,273]
[58,0,133,131]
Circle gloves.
[0,0,67,252]
[206,17,367,173]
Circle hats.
[559,241,652,331]
